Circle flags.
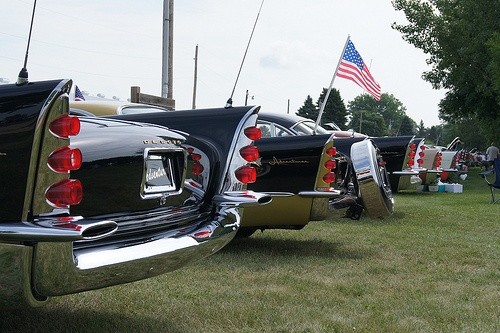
[335,37,382,102]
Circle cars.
[69,99,337,243]
[1,1,261,309]
[249,112,466,221]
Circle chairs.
[477,157,500,203]
[480,160,491,178]
[488,160,496,174]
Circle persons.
[485,142,499,174]
